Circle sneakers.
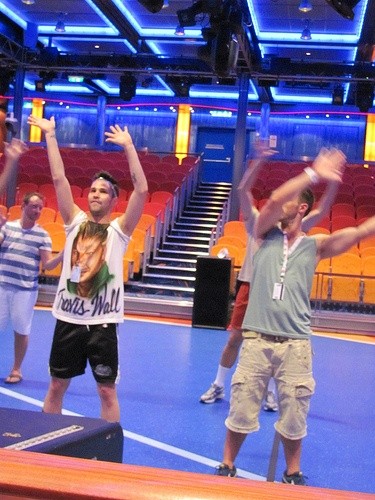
[282,470,307,485]
[264,392,279,411]
[215,463,237,477]
[200,383,226,404]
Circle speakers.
[192,256,232,330]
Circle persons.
[25,115,148,426]
[0,192,64,383]
[215,148,375,485]
[0,98,7,158]
[198,140,348,412]
[1,137,27,192]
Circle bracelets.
[304,166,319,185]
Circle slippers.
[4,374,22,384]
